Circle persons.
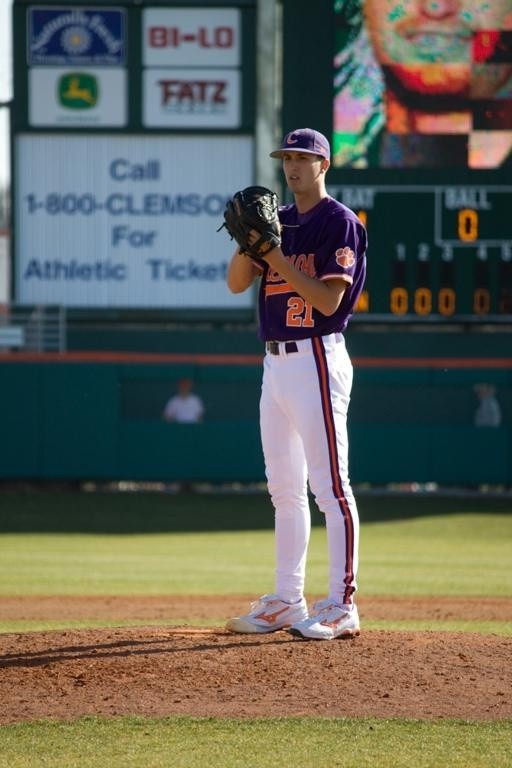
[214,124,371,641]
[336,1,510,172]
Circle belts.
[266,332,343,356]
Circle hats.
[269,127,331,161]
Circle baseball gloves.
[224,184,282,258]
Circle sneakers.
[223,596,308,635]
[288,600,361,641]
